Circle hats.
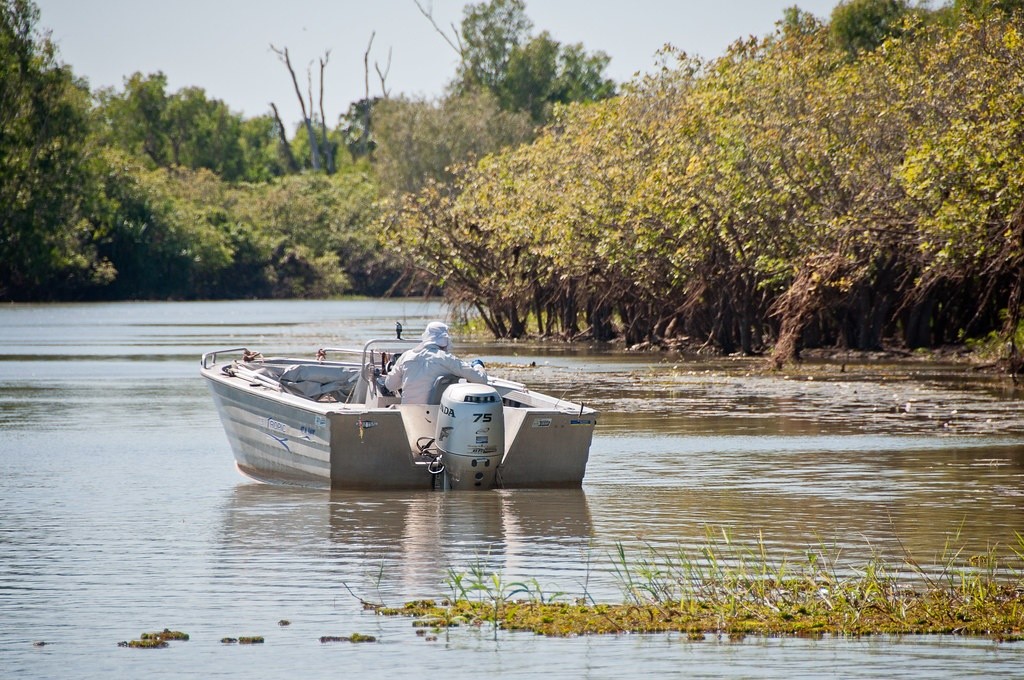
[421,322,451,348]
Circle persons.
[385,322,487,404]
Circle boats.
[199,340,601,489]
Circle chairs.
[428,374,459,405]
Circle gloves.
[471,360,485,369]
